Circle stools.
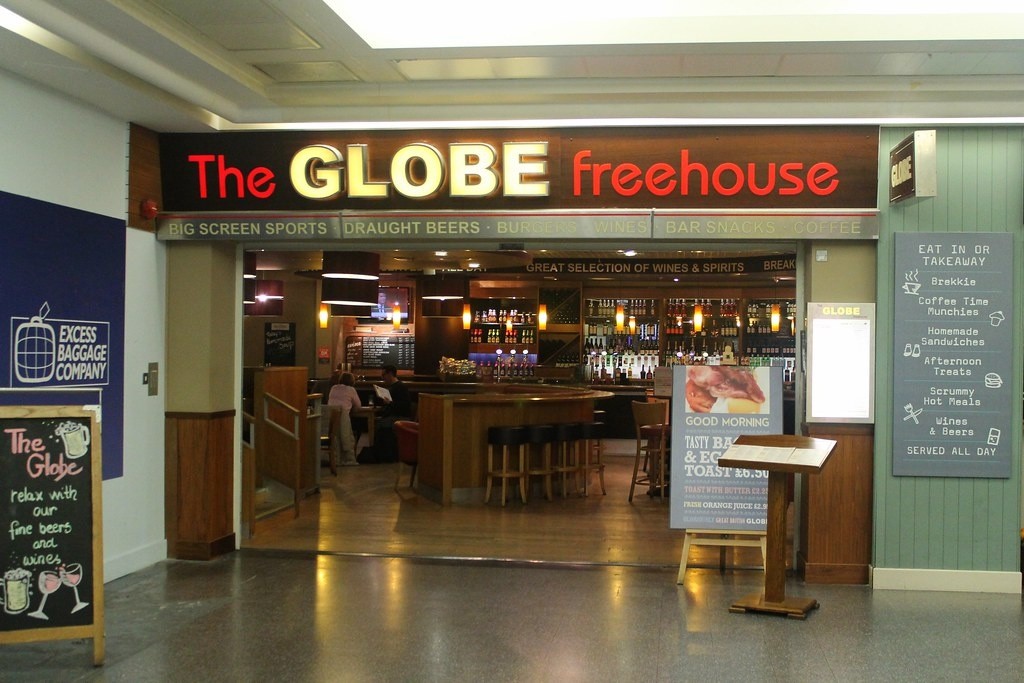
[551,423,582,499]
[628,400,671,506]
[578,422,607,496]
[484,424,528,507]
[524,424,556,502]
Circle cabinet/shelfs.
[470,287,795,385]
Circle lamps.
[321,277,379,307]
[392,257,400,329]
[693,305,703,332]
[770,277,780,333]
[616,305,624,331]
[331,304,371,319]
[538,303,546,330]
[422,275,465,301]
[463,269,472,330]
[242,268,285,317]
[321,250,380,281]
[628,317,636,335]
[318,303,328,329]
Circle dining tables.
[349,406,384,446]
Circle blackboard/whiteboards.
[264,322,296,367]
[0,403,105,645]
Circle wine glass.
[356,370,366,382]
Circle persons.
[373,364,414,462]
[327,372,361,465]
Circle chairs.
[394,419,420,487]
[320,411,341,477]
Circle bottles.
[469,329,483,343]
[504,329,517,343]
[664,298,739,367]
[522,329,535,344]
[555,352,580,368]
[525,363,529,375]
[500,361,505,375]
[550,310,580,324]
[530,363,535,376]
[519,363,523,376]
[493,361,498,375]
[474,309,537,323]
[513,364,518,376]
[585,299,659,379]
[487,329,500,343]
[745,300,797,385]
[486,361,492,374]
[479,361,485,375]
[507,364,512,376]
